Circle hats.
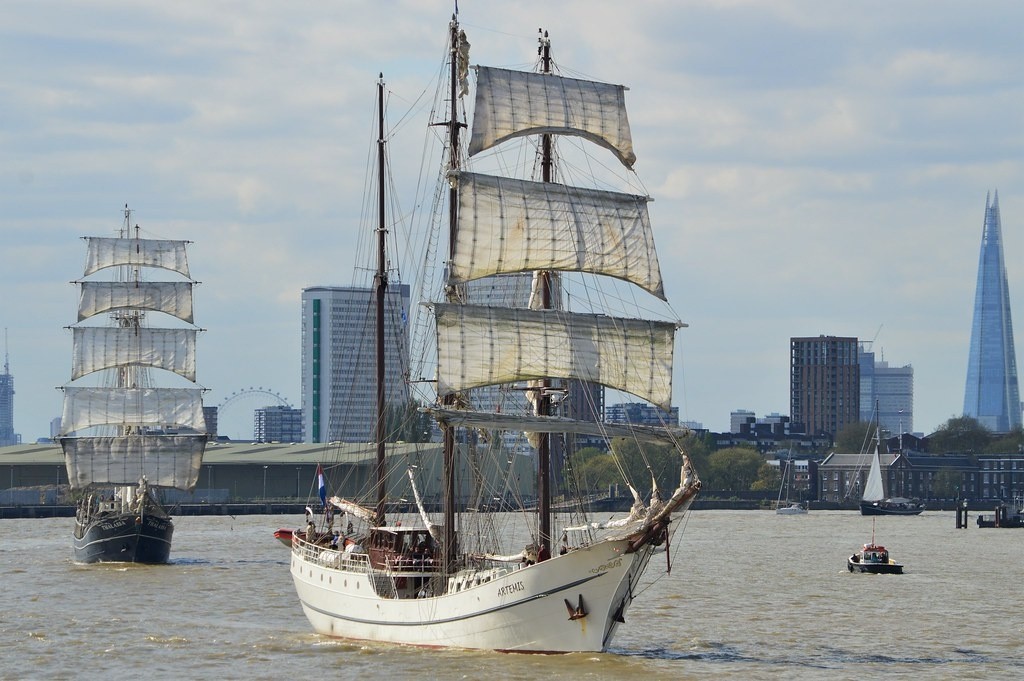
[309,520,312,522]
[334,531,339,533]
[397,521,401,526]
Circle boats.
[847,517,903,574]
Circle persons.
[1000,500,1007,519]
[852,553,887,564]
[880,503,921,511]
[78,478,144,518]
[306,522,568,571]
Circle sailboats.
[52,203,215,568]
[774,448,810,515]
[843,400,932,517]
[269,0,705,651]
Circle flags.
[317,463,328,507]
